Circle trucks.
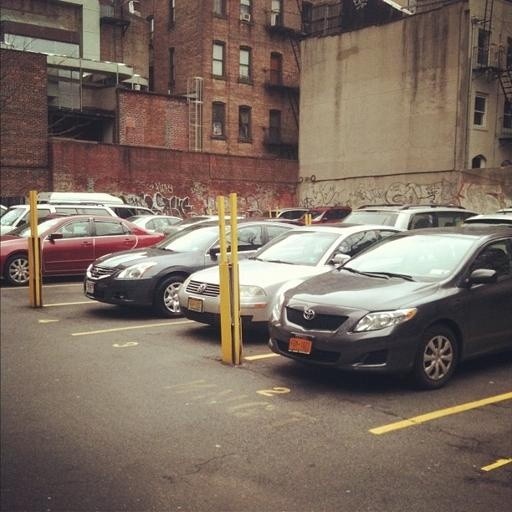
[36,191,156,219]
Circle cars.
[126,204,349,235]
[0,213,163,289]
[0,203,119,233]
[176,225,403,334]
[78,218,294,316]
[266,224,512,388]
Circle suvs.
[336,202,512,232]
